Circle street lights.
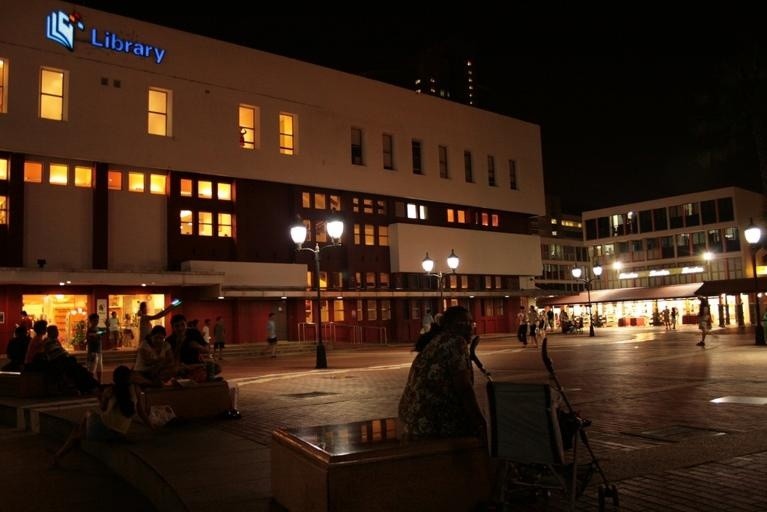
[743,219,766,347]
[421,249,459,313]
[571,261,602,338]
[289,207,344,368]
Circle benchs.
[137,380,230,422]
[271,418,481,512]
[0,371,46,398]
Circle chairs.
[486,382,618,510]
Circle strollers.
[469,334,619,508]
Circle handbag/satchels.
[147,405,176,428]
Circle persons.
[399,306,490,455]
[653,307,677,330]
[697,296,711,347]
[514,305,602,350]
[6,300,226,472]
[261,312,277,358]
